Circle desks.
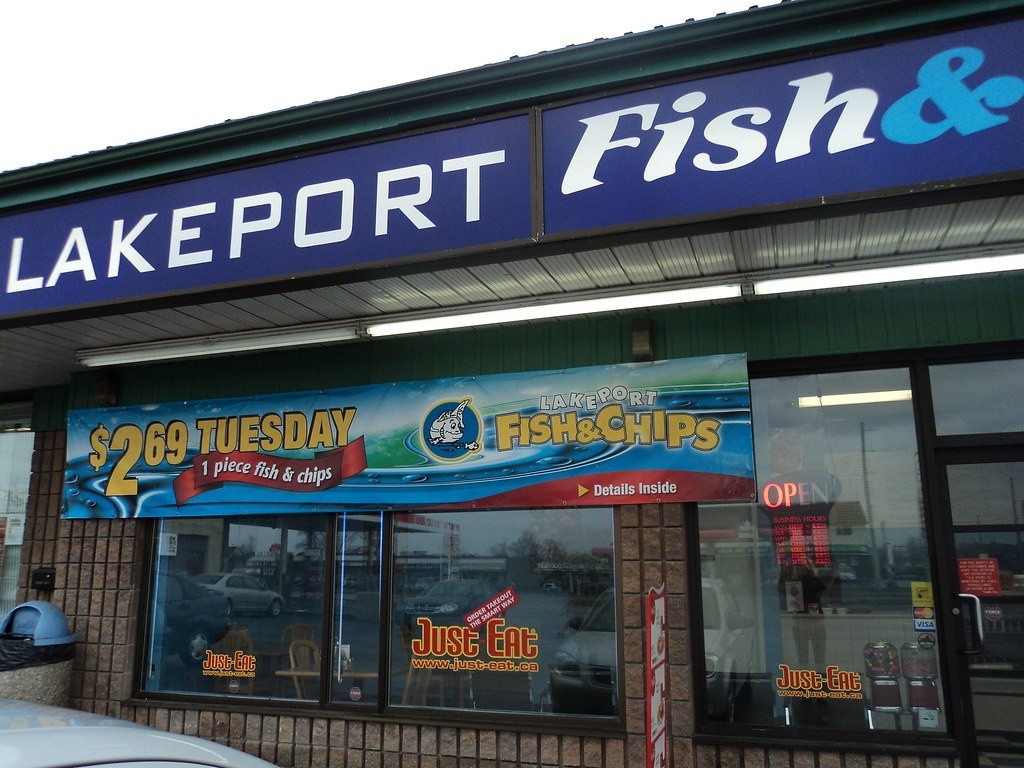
[250,650,291,657]
[275,667,410,702]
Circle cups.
[808,603,819,614]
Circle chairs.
[398,624,470,707]
[213,623,321,696]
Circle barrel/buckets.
[864,642,901,680]
[899,641,938,679]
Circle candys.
[863,646,899,678]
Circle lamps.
[75,235,1024,410]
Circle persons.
[779,551,826,664]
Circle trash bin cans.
[0,601,79,707]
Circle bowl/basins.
[822,608,832,613]
[836,608,847,614]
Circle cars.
[0,599,278,768]
[398,577,505,640]
[285,566,443,626]
[161,569,234,669]
[549,576,757,723]
[188,572,287,618]
[837,565,858,585]
